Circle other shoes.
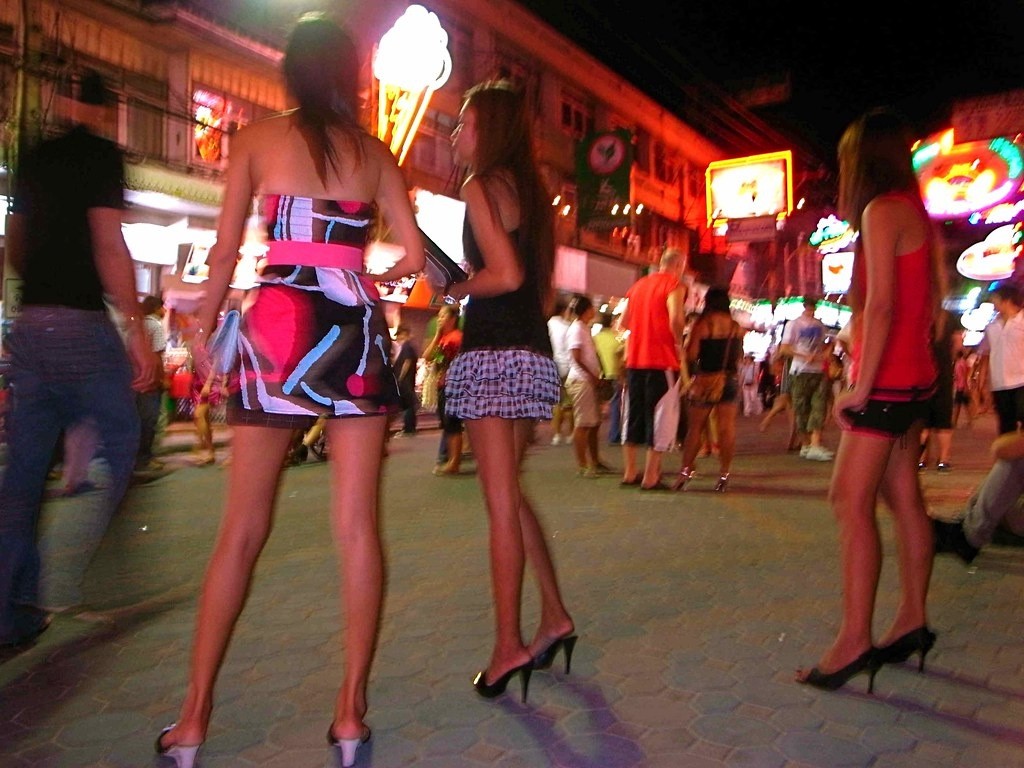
[393,428,417,440]
[564,436,573,445]
[697,440,721,462]
[917,462,928,475]
[136,459,182,484]
[936,460,953,474]
[925,514,980,568]
[549,433,562,446]
[431,466,460,478]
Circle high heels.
[530,630,578,677]
[326,719,372,768]
[796,645,882,693]
[669,467,696,492]
[874,625,937,672]
[472,658,531,705]
[714,473,730,492]
[153,723,205,768]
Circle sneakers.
[575,463,621,479]
[800,443,835,461]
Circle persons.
[289,416,326,458]
[444,81,579,704]
[49,295,166,498]
[0,62,155,647]
[933,433,1024,566]
[919,308,992,475]
[793,116,945,696]
[156,14,426,767]
[186,304,223,468]
[544,250,852,496]
[974,284,1024,436]
[422,304,474,477]
[393,325,418,437]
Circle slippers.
[640,479,671,492]
[617,472,644,486]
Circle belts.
[265,240,364,275]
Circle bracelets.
[122,315,145,332]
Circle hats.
[143,296,164,315]
